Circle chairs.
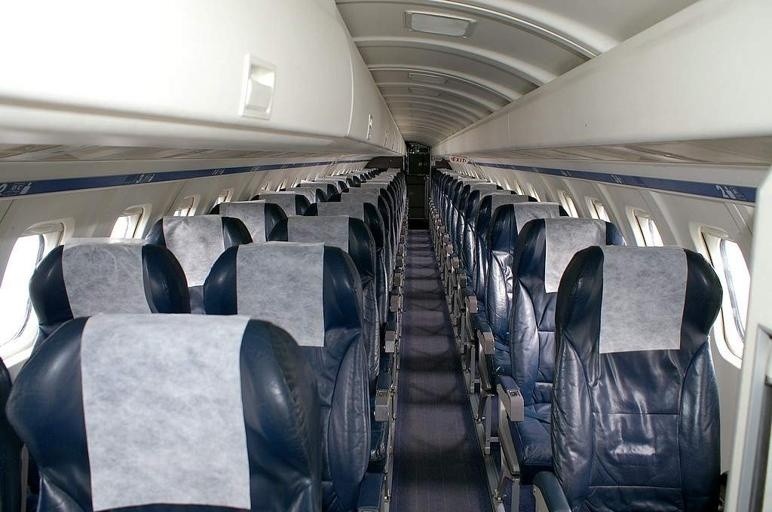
[423,166,731,512]
[3,168,411,512]
